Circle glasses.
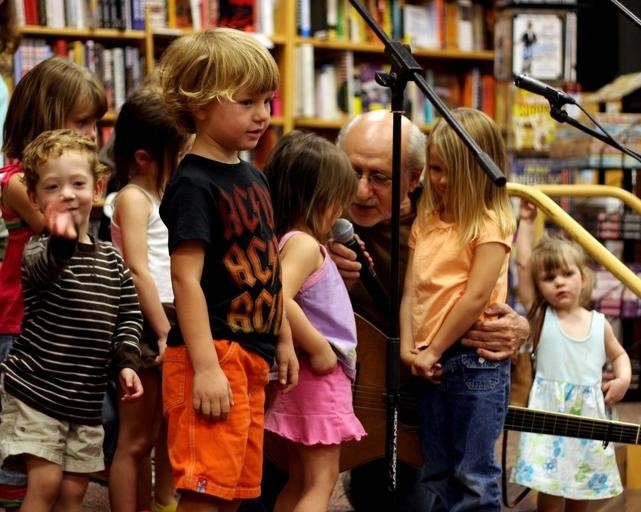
[352,167,414,190]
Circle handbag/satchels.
[505,350,533,407]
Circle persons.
[0,27,633,512]
[0,0,23,126]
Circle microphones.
[516,72,576,105]
[331,217,393,316]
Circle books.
[295,0,496,126]
[504,14,641,318]
[14,0,283,170]
